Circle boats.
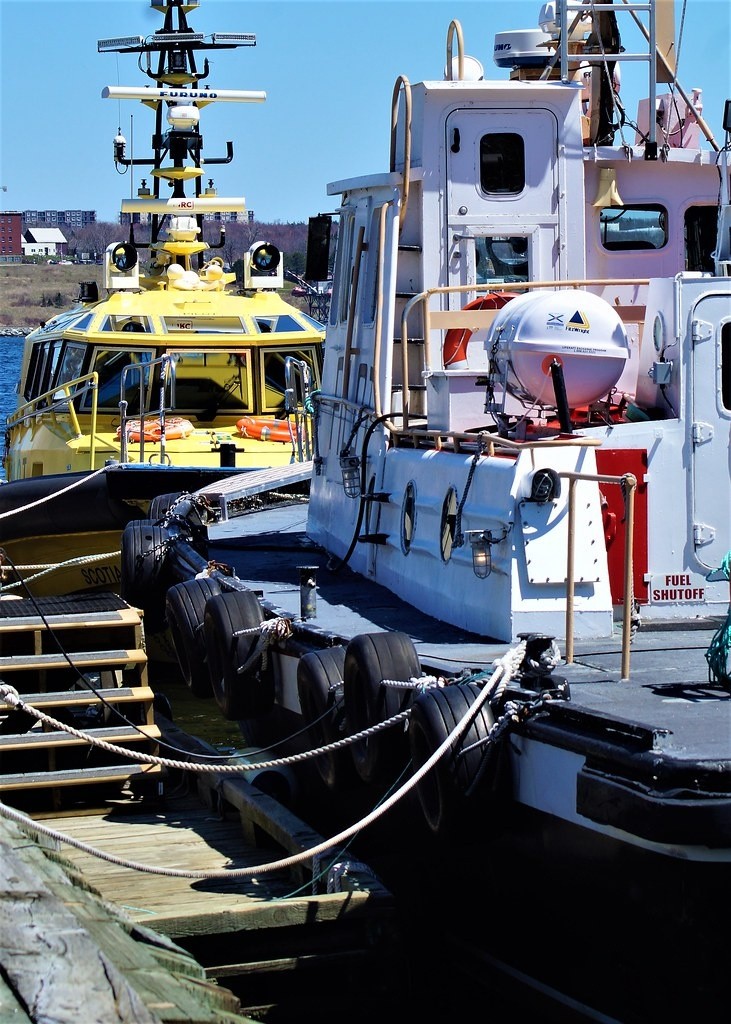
[0,1,331,600]
[121,1,730,1023]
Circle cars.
[22,256,104,266]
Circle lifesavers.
[122,492,185,529]
[164,577,222,692]
[237,418,306,442]
[296,646,346,786]
[204,592,267,719]
[120,524,162,607]
[343,633,422,780]
[443,291,523,371]
[409,687,513,835]
[116,418,193,442]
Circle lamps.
[466,529,501,580]
